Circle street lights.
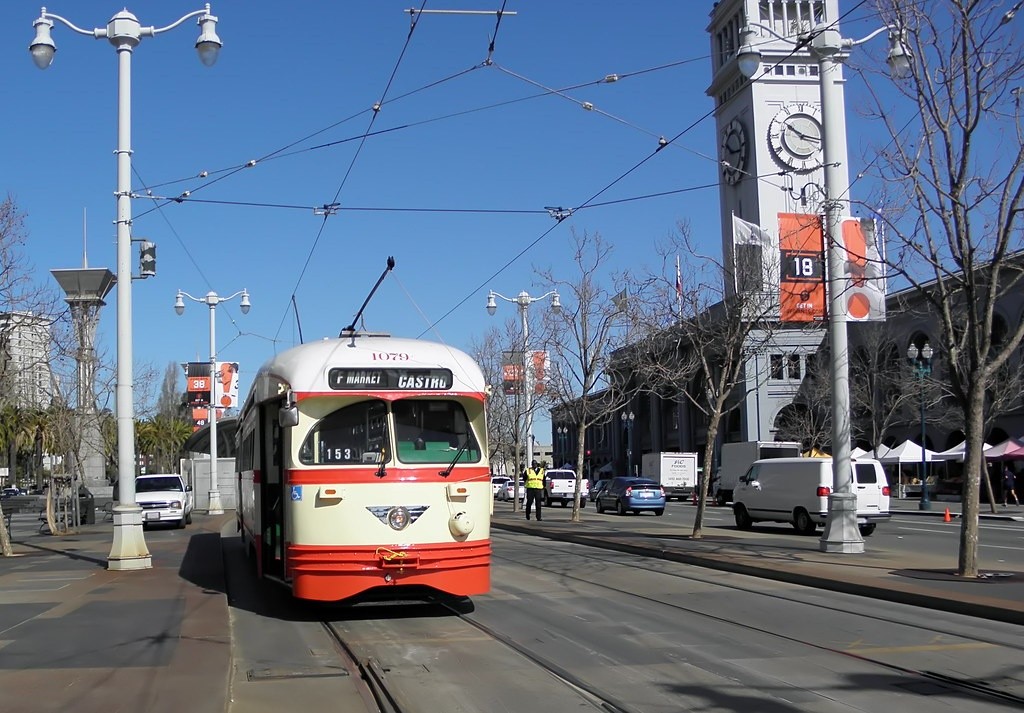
[737,18,911,554]
[486,288,562,510]
[708,390,719,471]
[28,4,226,571]
[557,427,568,468]
[906,344,933,509]
[172,285,252,516]
[621,411,636,476]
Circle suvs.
[491,476,511,496]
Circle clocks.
[721,118,748,184]
[769,104,826,169]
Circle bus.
[233,334,492,601]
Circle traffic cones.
[943,507,952,522]
[712,499,717,506]
[692,494,698,506]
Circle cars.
[590,480,609,501]
[497,480,525,502]
[595,477,666,516]
[0,487,26,502]
[134,474,193,529]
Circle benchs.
[2,502,113,538]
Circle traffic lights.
[586,449,590,460]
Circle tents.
[802,434,1024,503]
[560,461,613,480]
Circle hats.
[532,460,537,464]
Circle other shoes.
[537,518,542,521]
[527,517,530,520]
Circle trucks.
[712,441,803,506]
[636,452,698,501]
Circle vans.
[732,457,891,536]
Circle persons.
[523,460,546,520]
[1002,465,1019,506]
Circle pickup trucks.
[542,470,590,508]
